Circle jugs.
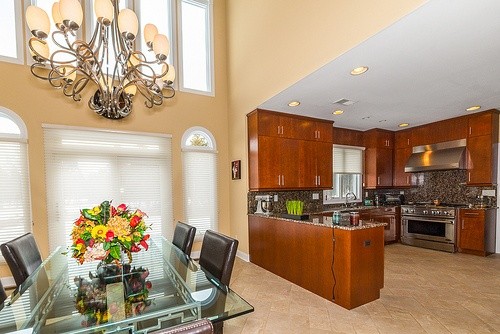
[474,194,492,209]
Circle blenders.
[254,193,274,215]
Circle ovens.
[399,215,458,253]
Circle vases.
[99,251,124,283]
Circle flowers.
[60,200,153,271]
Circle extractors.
[403,138,468,173]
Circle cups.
[332,210,341,224]
[349,211,360,226]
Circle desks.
[0,235,254,334]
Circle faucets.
[345,192,357,208]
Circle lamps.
[24,1,176,120]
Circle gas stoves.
[400,198,472,219]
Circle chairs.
[144,220,197,294]
[0,280,17,334]
[128,229,240,334]
[0,232,79,319]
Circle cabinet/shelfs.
[455,205,496,257]
[362,127,394,190]
[246,108,335,191]
[321,204,402,244]
[392,128,424,189]
[464,109,500,188]
